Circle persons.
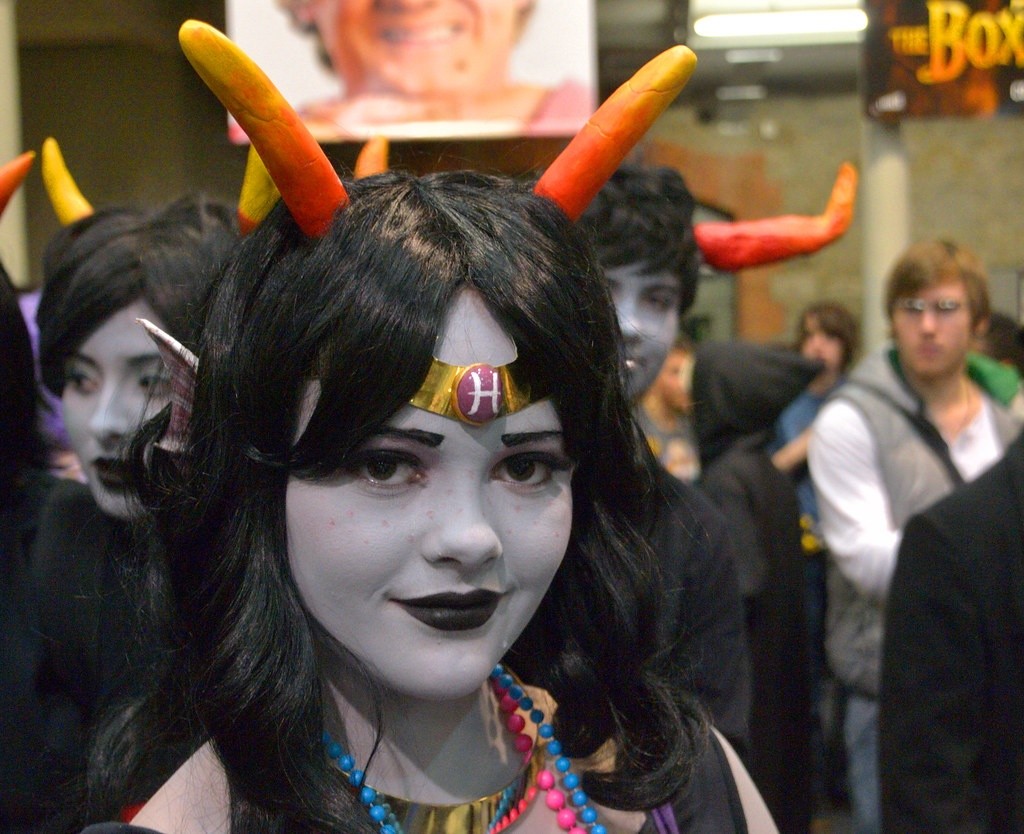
[804,236,1016,833]
[0,133,299,834]
[615,318,714,506]
[749,283,861,767]
[225,0,599,133]
[83,13,782,833]
[875,398,1024,827]
[518,139,860,752]
